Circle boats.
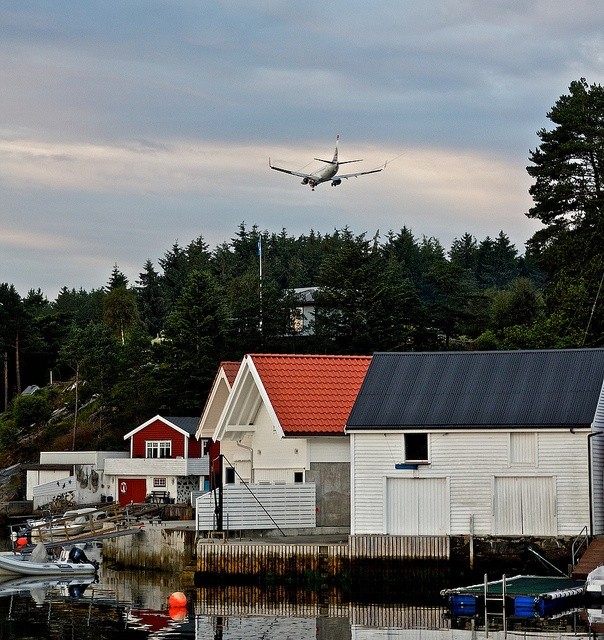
[0,575,98,608]
[63,508,107,531]
[31,526,86,537]
[0,542,98,575]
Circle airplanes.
[269,135,387,191]
